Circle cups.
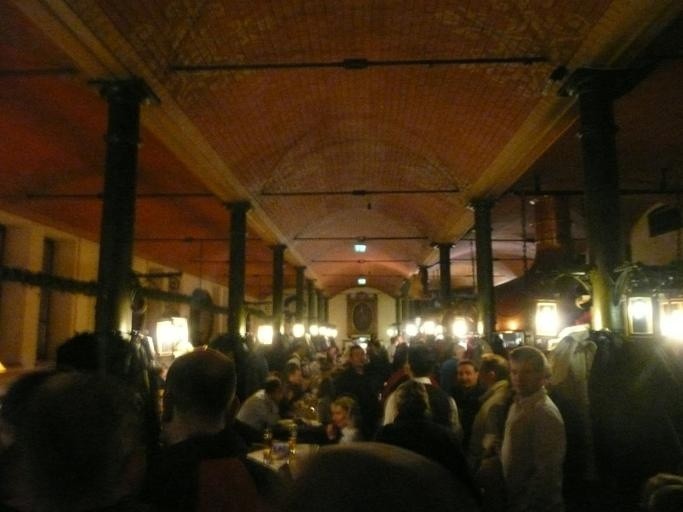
[261,428,295,466]
